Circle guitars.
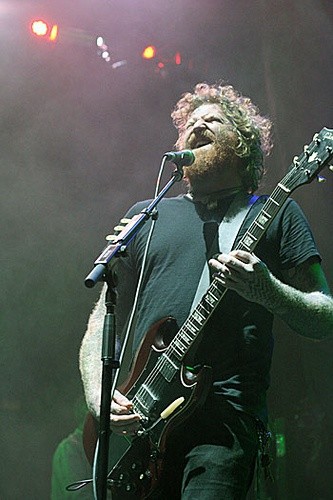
[82,126,333,499]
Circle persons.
[83,80,332,500]
[49,408,100,500]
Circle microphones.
[163,148,195,178]
